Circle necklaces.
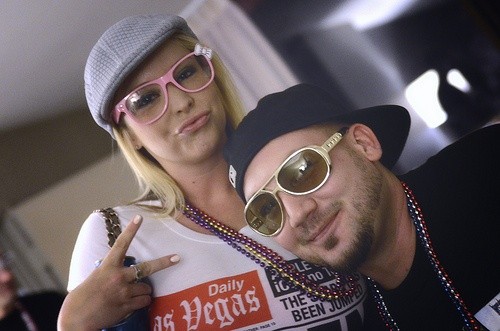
[15,303,35,331]
[175,198,355,301]
[365,179,482,331]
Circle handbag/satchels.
[92,208,151,330]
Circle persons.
[58,13,373,330]
[1,256,69,331]
[228,83,500,331]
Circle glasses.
[243,127,348,237]
[112,44,215,125]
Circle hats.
[223,80,412,202]
[84,13,198,140]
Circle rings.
[128,264,144,283]
